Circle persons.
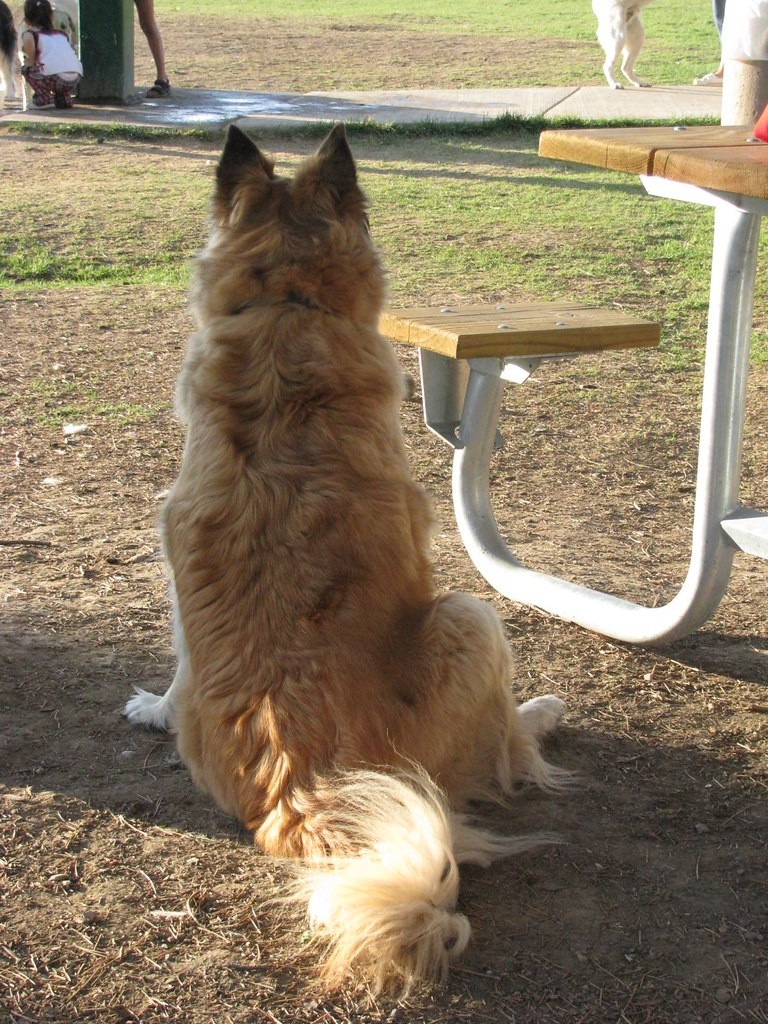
[22,0,82,110]
[693,0,726,85]
[137,0,173,100]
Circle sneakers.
[693,73,723,87]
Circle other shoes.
[27,101,55,109]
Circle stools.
[379,297,663,584]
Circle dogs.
[0,0,79,111]
[124,118,571,994]
[591,0,653,90]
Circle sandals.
[145,79,171,98]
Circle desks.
[488,128,768,646]
